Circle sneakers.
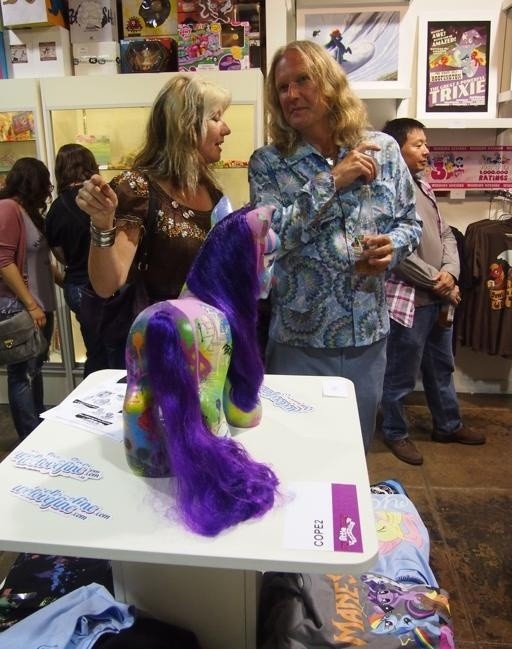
[380,427,424,468]
[430,421,487,447]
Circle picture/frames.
[296,6,408,87]
[418,14,498,117]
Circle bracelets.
[26,303,41,311]
[90,215,118,248]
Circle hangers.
[489,196,512,222]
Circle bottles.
[351,183,381,274]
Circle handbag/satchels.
[0,304,52,370]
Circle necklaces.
[322,146,341,164]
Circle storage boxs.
[0,0,179,78]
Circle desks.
[0,370,378,649]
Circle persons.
[0,155,66,443]
[80,74,235,381]
[46,143,103,352]
[247,39,424,459]
[379,118,485,466]
